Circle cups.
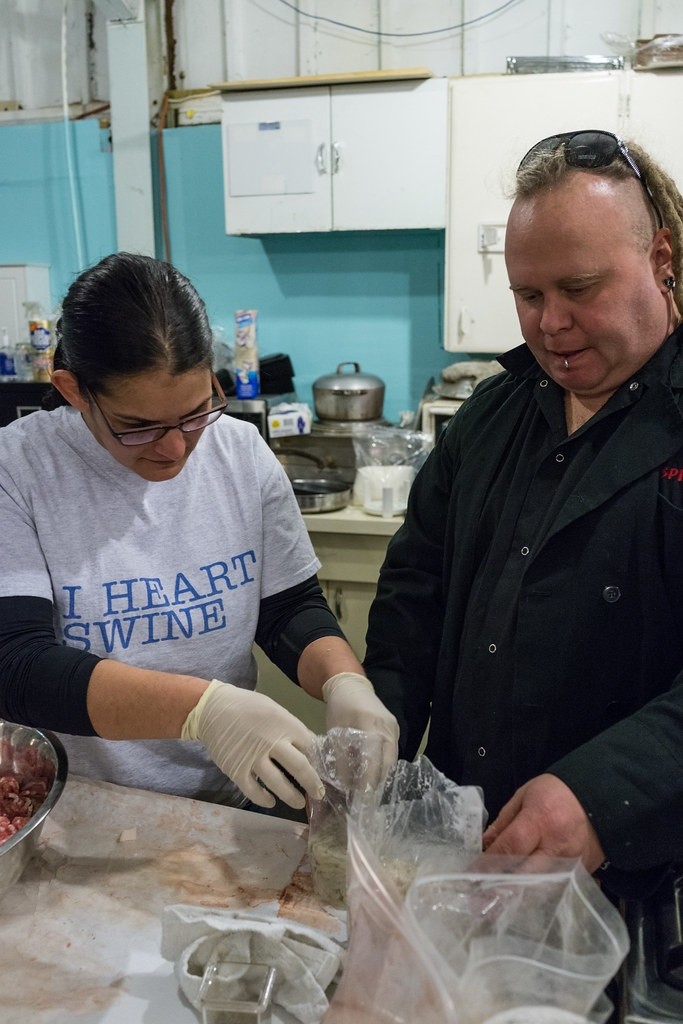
[196,959,276,1024]
[13,341,35,383]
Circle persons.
[0,255,401,821]
[364,131,683,1023]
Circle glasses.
[515,129,663,231]
[74,370,229,447]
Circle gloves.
[321,671,402,810]
[178,677,334,812]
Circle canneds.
[0,318,55,383]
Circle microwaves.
[421,396,465,457]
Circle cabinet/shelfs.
[246,584,376,734]
[221,80,444,234]
[446,72,683,354]
[1,263,50,349]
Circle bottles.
[0,325,16,382]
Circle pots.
[311,360,385,422]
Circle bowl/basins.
[0,718,70,903]
[291,477,351,515]
